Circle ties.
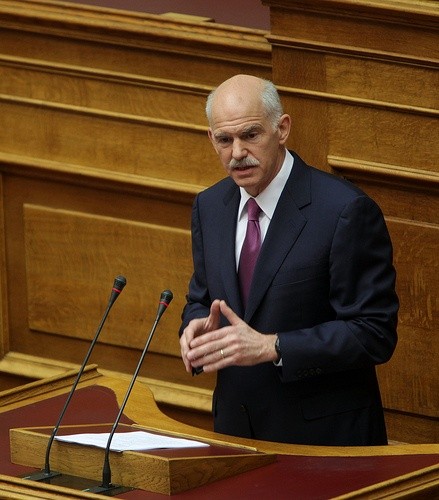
[238,198,263,312]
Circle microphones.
[101,291,173,488]
[44,276,127,476]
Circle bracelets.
[275,333,281,364]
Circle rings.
[220,348,225,358]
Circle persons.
[177,74,400,446]
